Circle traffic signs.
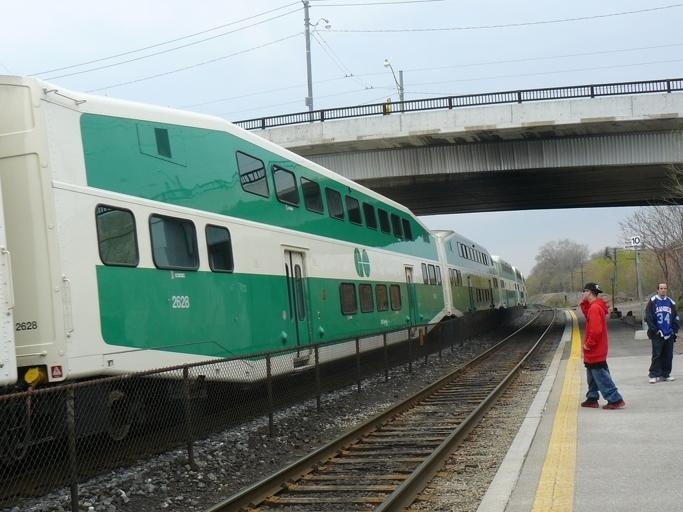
[631,236,641,246]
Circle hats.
[584,282,603,293]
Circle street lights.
[303,0,332,121]
[383,58,404,112]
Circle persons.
[644,281,681,383]
[579,281,626,411]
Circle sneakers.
[582,402,598,408]
[663,376,675,381]
[603,400,624,408]
[649,377,660,382]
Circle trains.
[0,74,528,478]
